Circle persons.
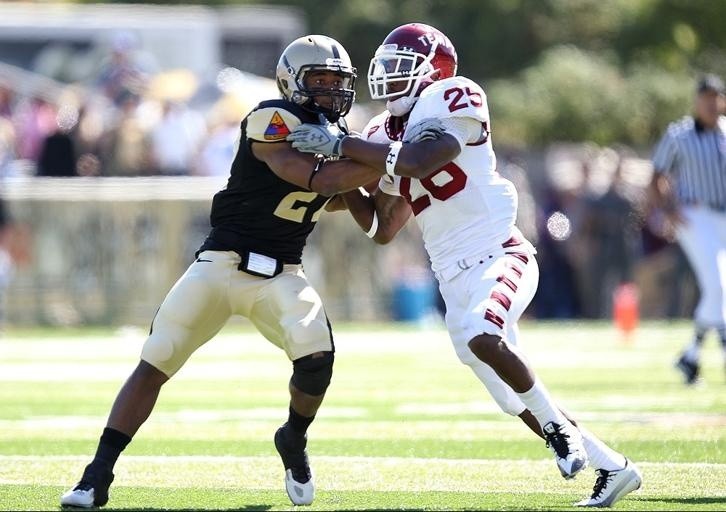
[286,23,641,508]
[1,49,243,175]
[498,146,700,324]
[60,34,447,511]
[650,75,726,380]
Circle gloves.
[286,113,347,158]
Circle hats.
[696,71,724,94]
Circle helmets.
[275,34,357,120]
[367,22,459,116]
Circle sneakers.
[678,354,702,386]
[275,421,314,505]
[60,463,115,508]
[547,413,588,481]
[572,458,641,507]
